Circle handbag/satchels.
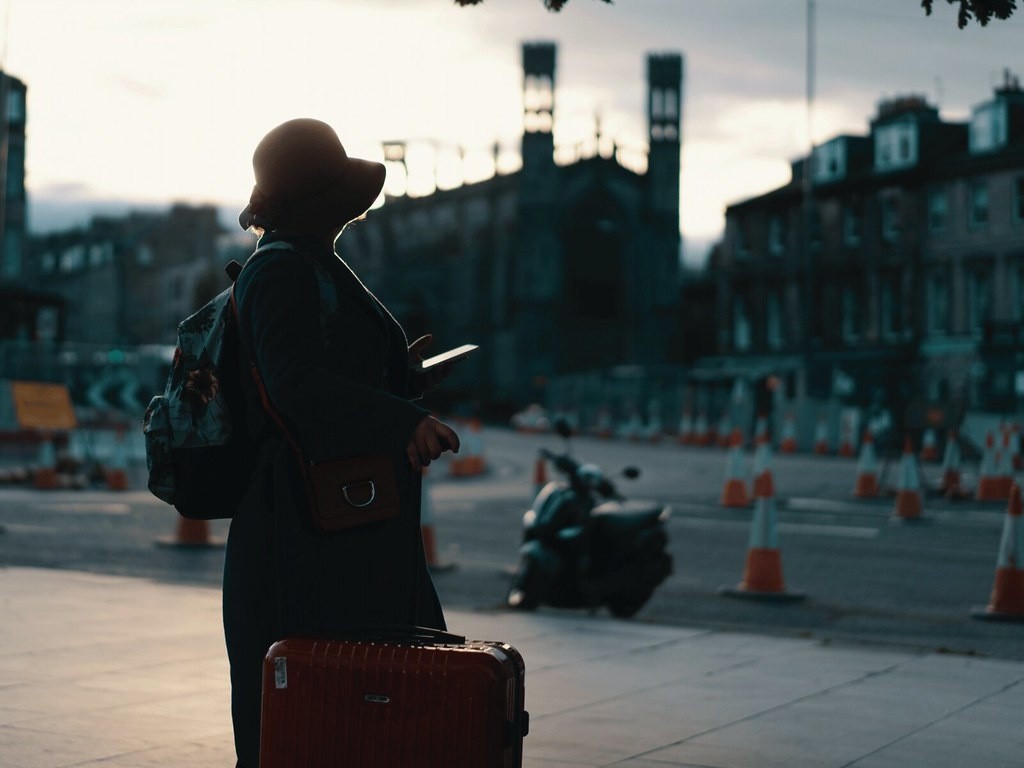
[305,453,401,533]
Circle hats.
[239,121,386,231]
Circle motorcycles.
[506,415,675,621]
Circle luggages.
[259,625,529,768]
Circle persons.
[218,118,466,768]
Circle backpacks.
[143,237,293,520]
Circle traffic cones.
[814,416,832,461]
[444,418,489,478]
[916,412,1024,501]
[35,427,62,491]
[850,427,886,503]
[893,436,929,524]
[751,415,790,510]
[839,411,860,458]
[420,464,461,574]
[101,421,134,492]
[529,456,550,498]
[153,515,228,549]
[511,403,732,448]
[971,483,1024,623]
[782,417,800,456]
[716,467,807,602]
[722,418,751,509]
